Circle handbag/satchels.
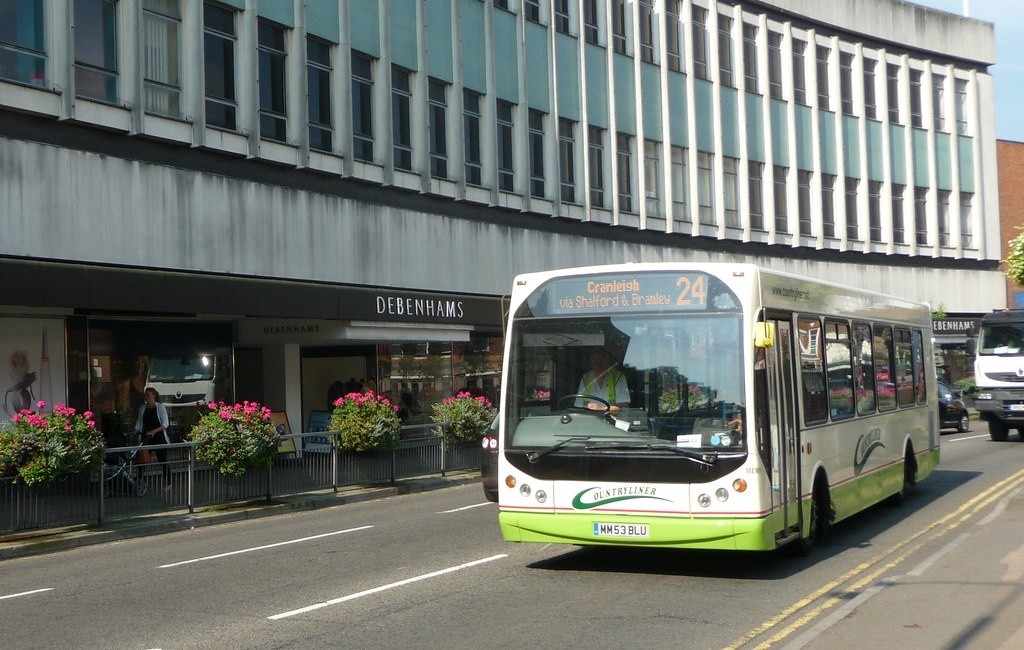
[135,449,150,464]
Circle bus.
[497,262,941,556]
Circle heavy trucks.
[143,353,232,442]
[974,309,1024,441]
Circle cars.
[936,381,969,433]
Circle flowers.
[657,384,704,415]
[520,389,550,414]
[430,390,498,450]
[187,401,280,476]
[323,390,402,454]
[0,400,107,488]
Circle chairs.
[794,361,915,420]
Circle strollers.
[78,432,147,497]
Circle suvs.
[481,398,551,502]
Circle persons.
[574,350,630,415]
[360,377,391,396]
[327,378,361,413]
[131,388,174,493]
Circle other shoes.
[160,481,171,492]
